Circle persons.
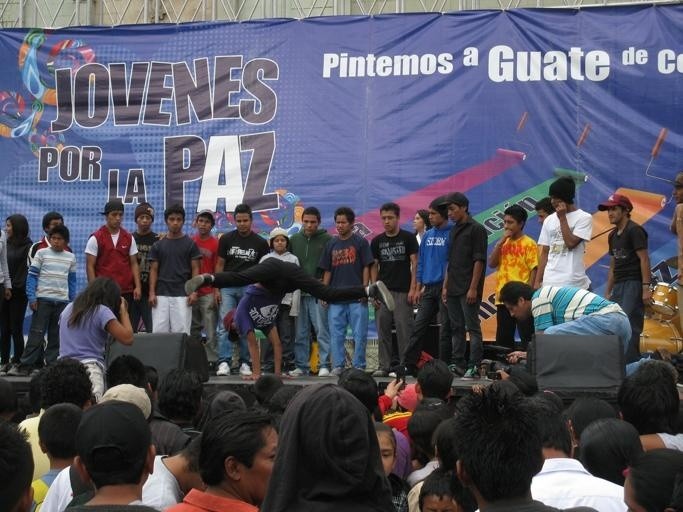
[0,170,683,508]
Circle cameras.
[396,366,406,390]
[487,373,501,380]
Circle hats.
[598,194,633,211]
[223,309,239,342]
[77,400,151,468]
[98,200,124,214]
[438,192,469,211]
[135,203,154,221]
[197,210,214,227]
[270,227,288,239]
[100,383,152,420]
[397,384,417,410]
[549,176,575,204]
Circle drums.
[645,282,678,316]
[639,319,683,353]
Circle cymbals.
[666,256,680,269]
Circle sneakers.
[369,280,395,312]
[217,361,230,375]
[291,367,408,376]
[461,369,480,381]
[185,274,212,296]
[651,349,663,360]
[449,364,465,377]
[240,363,252,376]
[0,364,40,376]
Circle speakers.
[528,332,626,390]
[105,331,209,383]
